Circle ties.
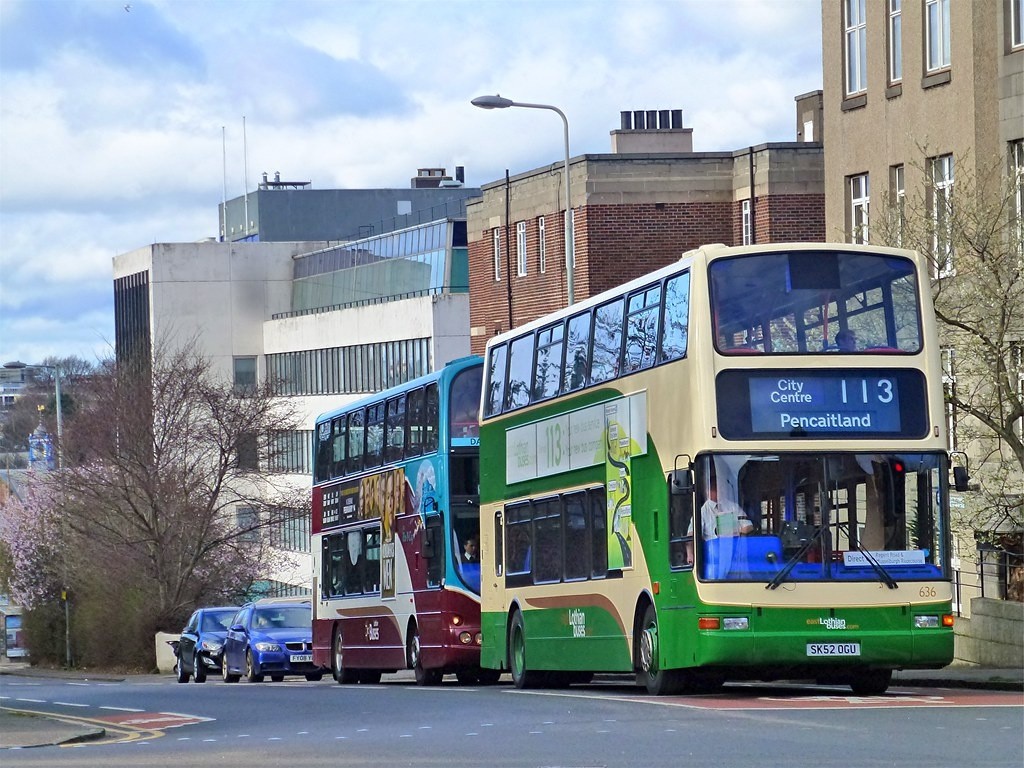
[470,556,474,561]
[716,504,723,513]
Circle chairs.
[327,443,435,479]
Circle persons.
[835,328,858,352]
[466,408,485,436]
[460,537,480,562]
[686,476,754,566]
[358,467,404,540]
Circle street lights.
[3,360,71,672]
[470,94,574,308]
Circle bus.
[479,243,971,698]
[311,351,597,687]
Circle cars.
[177,607,256,684]
[220,601,322,682]
